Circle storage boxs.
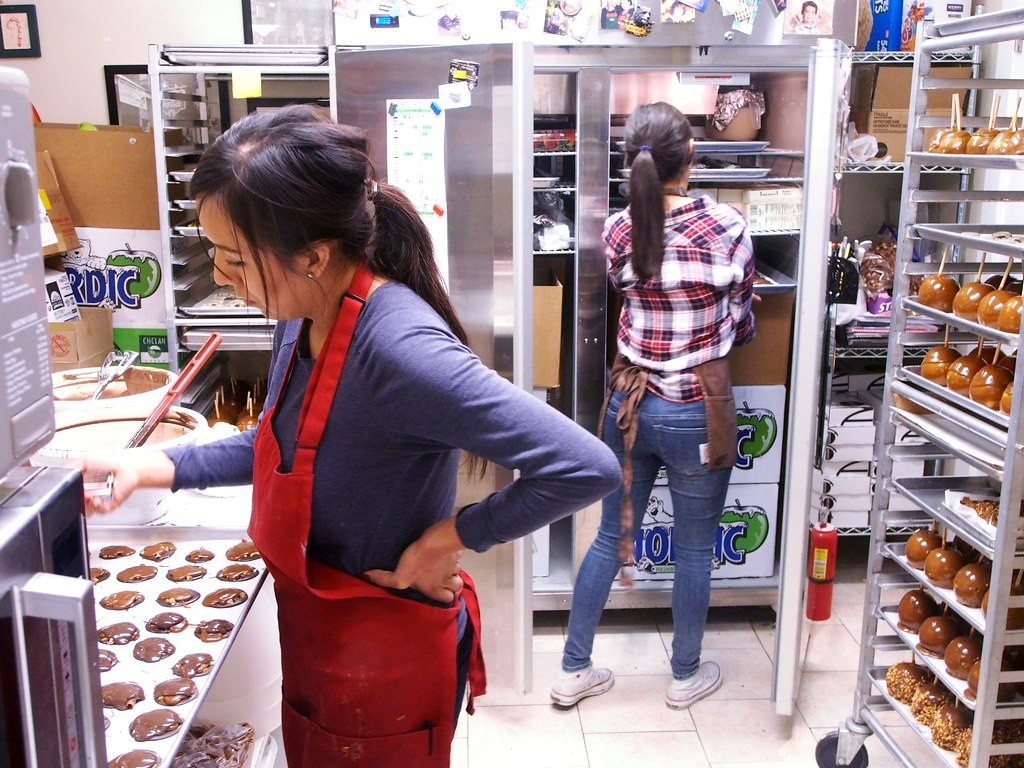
[728,289,795,385]
[652,385,788,483]
[34,122,162,229]
[49,306,114,371]
[621,485,778,578]
[45,259,81,323]
[61,228,172,371]
[850,64,971,164]
[37,147,82,256]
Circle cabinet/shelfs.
[814,7,1024,768]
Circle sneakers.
[665,661,723,710]
[550,662,614,706]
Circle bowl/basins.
[28,406,207,523]
[53,366,184,415]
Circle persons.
[73,106,623,768]
[552,102,756,711]
[793,0,828,33]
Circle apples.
[76,123,97,130]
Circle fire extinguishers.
[806,505,838,622]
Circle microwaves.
[0,456,109,768]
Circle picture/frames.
[245,95,329,115]
[103,65,231,138]
[0,4,42,58]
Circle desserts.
[207,362,267,433]
[884,122,1024,768]
[84,537,262,768]
